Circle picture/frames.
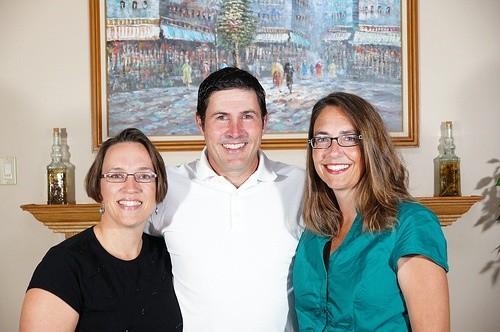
[89,1,418,150]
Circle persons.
[144,65,308,332]
[292,92,451,332]
[19,127,183,331]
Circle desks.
[18,193,485,240]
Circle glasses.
[100,171,158,183]
[307,134,362,149]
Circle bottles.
[439,121,461,197]
[46,128,67,204]
